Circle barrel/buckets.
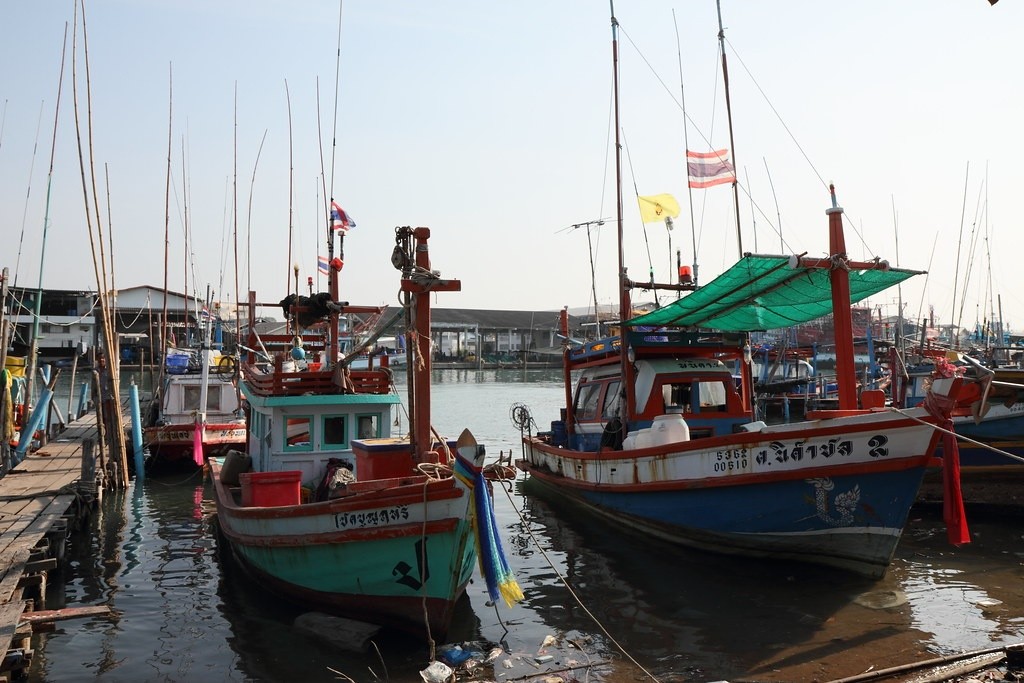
[213,355,235,366]
[623,431,638,450]
[220,450,252,484]
[635,428,651,449]
[168,358,188,372]
[651,414,690,447]
[551,421,566,445]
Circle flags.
[687,149,736,189]
[318,255,330,275]
[202,305,216,321]
[637,194,679,224]
[330,200,356,232]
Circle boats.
[140,0,1024,637]
